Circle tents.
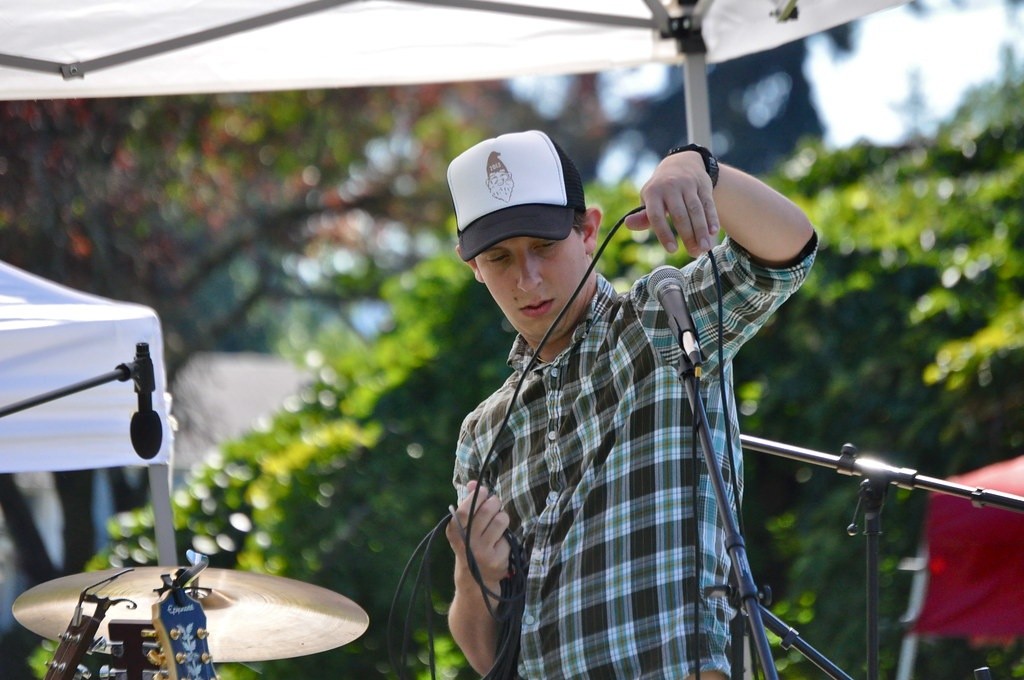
[0,0,927,680]
[0,252,181,574]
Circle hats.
[445,129,587,263]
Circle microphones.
[129,343,163,461]
[645,264,708,367]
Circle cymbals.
[10,563,371,665]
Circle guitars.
[29,565,137,680]
[139,547,223,680]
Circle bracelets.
[666,142,720,185]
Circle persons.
[440,124,819,680]
